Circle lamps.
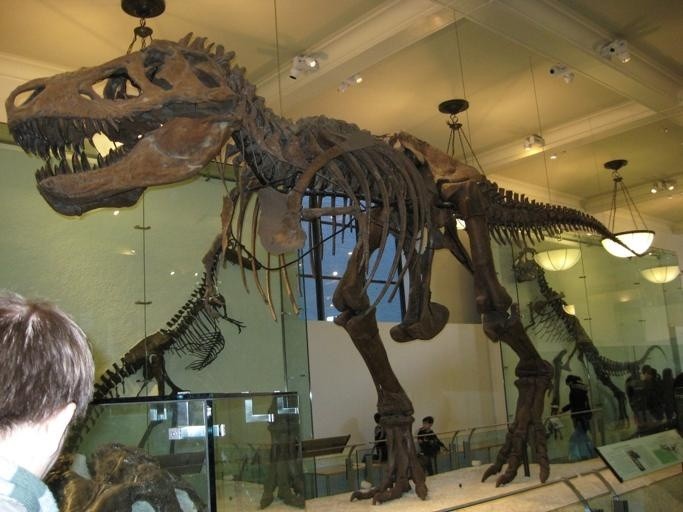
[602,159,655,259]
[438,99,485,231]
[640,264,680,284]
[337,73,362,92]
[650,178,674,194]
[523,134,545,151]
[532,247,582,272]
[600,39,631,63]
[288,55,317,80]
[548,63,575,85]
[562,305,575,315]
[92,0,165,158]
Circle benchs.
[304,438,505,498]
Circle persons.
[562,374,593,460]
[1,294,96,512]
[626,365,683,433]
[374,413,388,464]
[414,415,450,476]
